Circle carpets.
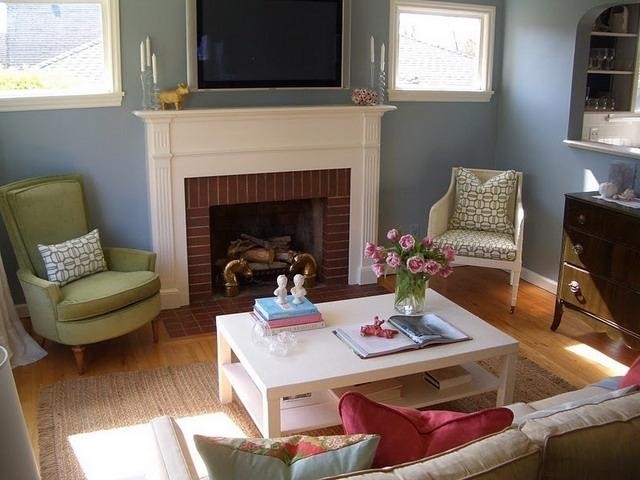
[37,356,581,480]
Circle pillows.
[447,167,517,235]
[186,430,379,480]
[618,354,639,390]
[338,390,516,465]
[37,229,108,287]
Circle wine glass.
[589,47,616,70]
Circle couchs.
[146,366,639,480]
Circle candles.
[370,35,375,63]
[140,41,146,71]
[380,42,385,71]
[152,52,157,84]
[146,35,151,66]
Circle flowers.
[364,228,452,304]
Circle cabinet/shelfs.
[582,3,640,113]
[550,188,639,347]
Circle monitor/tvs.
[196,0,343,89]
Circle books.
[332,308,476,362]
[252,295,324,337]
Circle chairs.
[1,173,163,374]
[424,166,526,314]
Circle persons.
[291,272,307,305]
[273,272,288,307]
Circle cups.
[585,87,616,111]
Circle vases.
[388,265,425,312]
